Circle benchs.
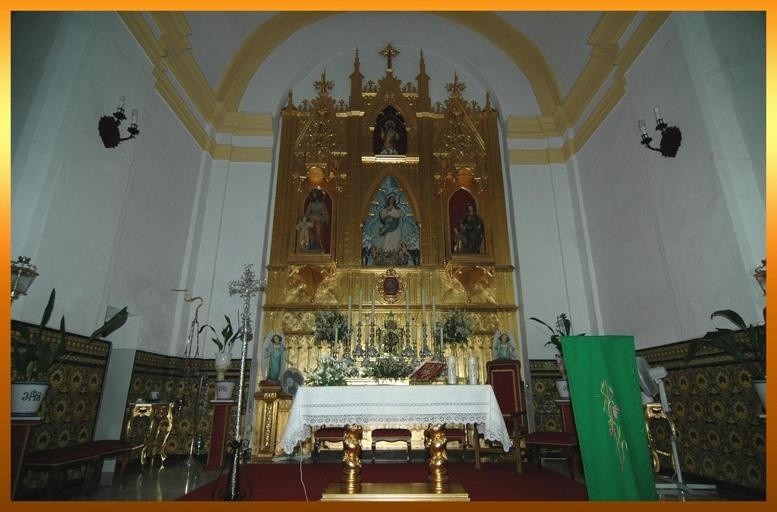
[22,438,144,501]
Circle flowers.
[303,355,349,386]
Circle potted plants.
[10,283,129,416]
[686,304,766,415]
[196,312,244,373]
[528,312,587,399]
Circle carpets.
[177,464,589,501]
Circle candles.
[334,283,445,356]
[116,94,126,114]
[639,118,648,139]
[131,109,137,127]
[655,105,662,123]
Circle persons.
[380,125,401,149]
[494,333,515,358]
[304,190,330,248]
[379,191,405,249]
[458,203,483,252]
[265,335,286,381]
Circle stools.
[312,426,349,462]
[368,428,413,463]
[526,430,579,480]
[423,427,466,464]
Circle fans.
[280,366,305,402]
[633,355,717,491]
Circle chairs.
[474,358,530,477]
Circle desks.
[279,383,515,501]
[640,402,680,486]
[123,400,177,469]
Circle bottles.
[468,357,477,383]
[447,355,456,384]
